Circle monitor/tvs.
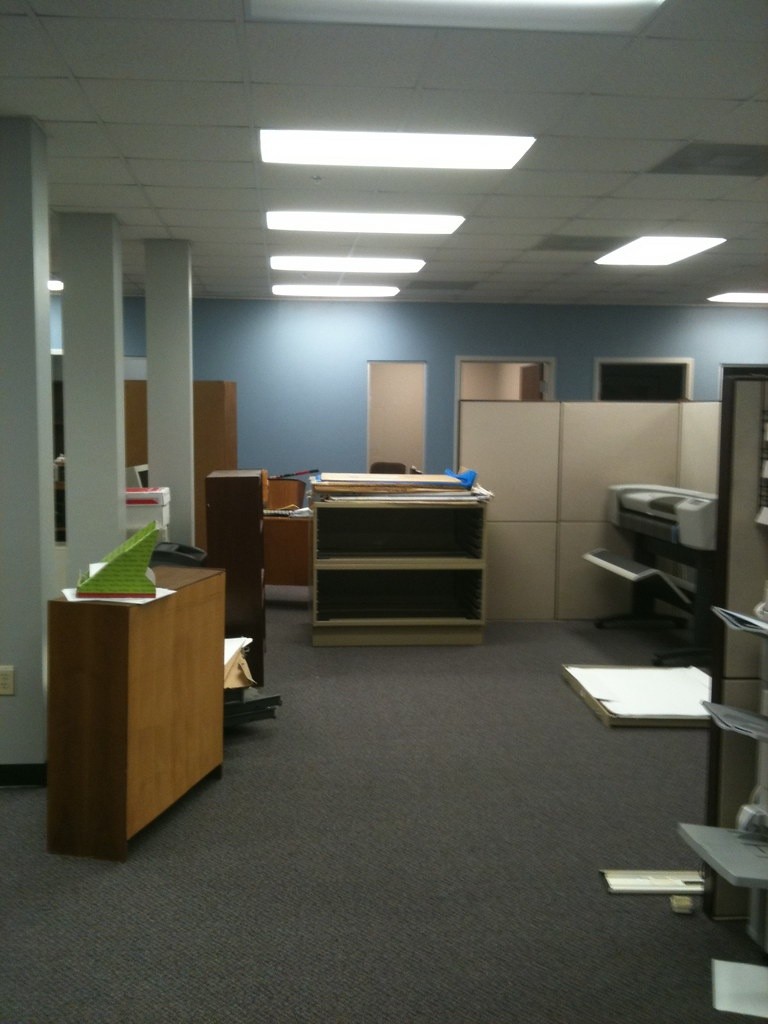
[126,463,148,488]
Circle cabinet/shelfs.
[458,400,721,622]
[124,379,237,522]
[678,364,766,954]
[47,566,226,862]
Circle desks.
[310,477,486,645]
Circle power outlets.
[0,666,14,696]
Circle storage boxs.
[126,487,170,528]
